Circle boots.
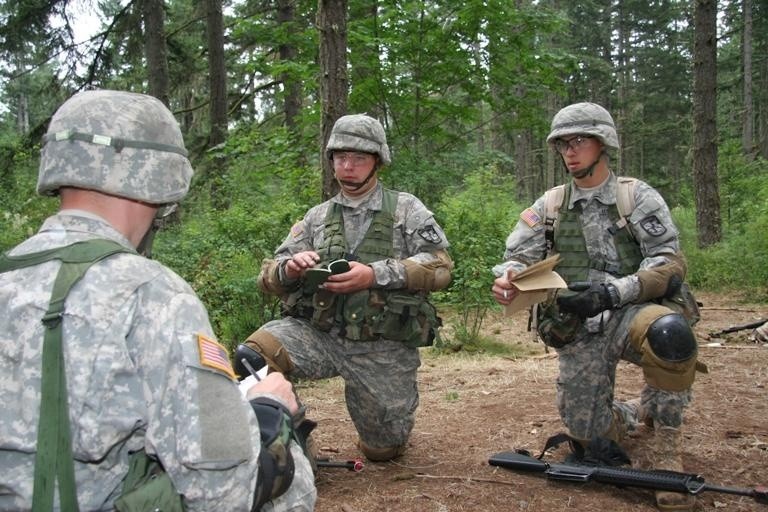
[652,432,696,511]
[626,398,654,427]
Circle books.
[304,258,351,293]
[503,253,568,320]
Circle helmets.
[545,101,619,153]
[326,114,391,165]
[35,90,193,205]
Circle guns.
[317,458,364,471]
[710,319,766,336]
[488,450,767,505]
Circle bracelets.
[604,281,619,309]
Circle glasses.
[330,153,368,165]
[555,137,590,151]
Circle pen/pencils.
[241,358,262,382]
[504,270,508,299]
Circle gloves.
[557,281,618,318]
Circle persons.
[234,113,454,463]
[491,103,700,511]
[0,89,319,511]
